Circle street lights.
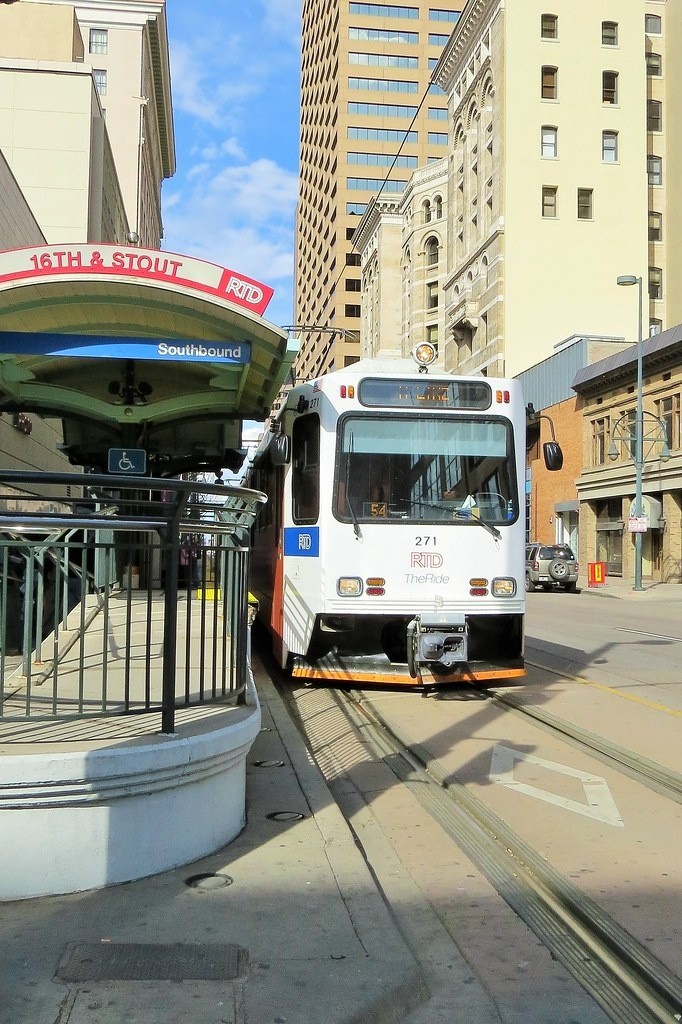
[616,275,647,592]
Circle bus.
[231,341,564,688]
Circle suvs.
[526,543,581,593]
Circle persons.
[179,540,201,589]
[353,483,384,516]
[441,484,464,501]
